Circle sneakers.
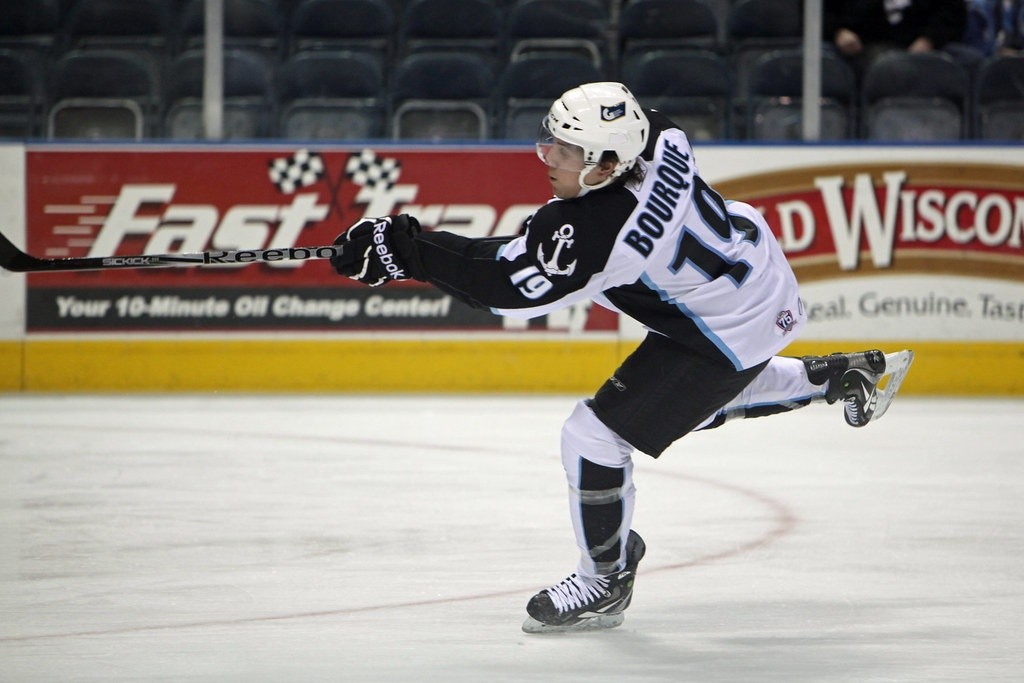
[522,530,645,632]
[802,349,915,427]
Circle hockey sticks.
[0,230,352,274]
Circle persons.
[822,1,970,103]
[329,80,914,634]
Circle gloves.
[329,214,427,287]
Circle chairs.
[0,0,1024,144]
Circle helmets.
[542,81,650,173]
